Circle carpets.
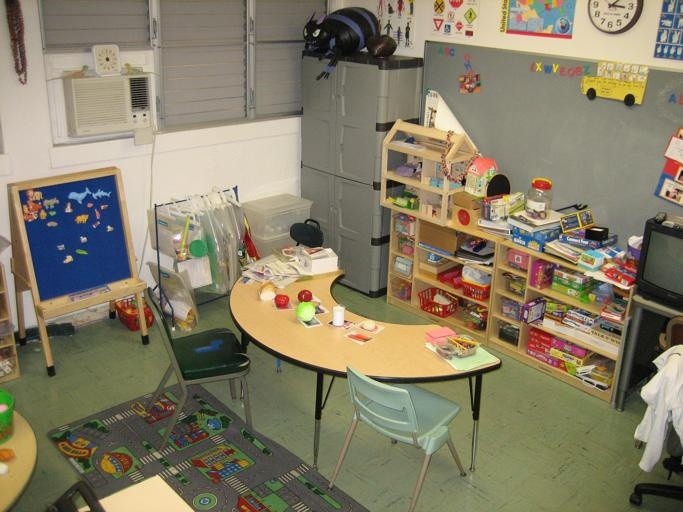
[46,378,379,512]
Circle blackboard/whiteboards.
[11,166,139,307]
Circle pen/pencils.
[447,335,480,351]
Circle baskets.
[462,278,491,301]
[418,288,459,317]
[114,296,154,332]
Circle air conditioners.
[61,76,161,138]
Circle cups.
[332,305,345,326]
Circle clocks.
[88,44,125,78]
[588,0,644,35]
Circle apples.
[297,290,312,302]
[296,301,315,322]
[275,294,289,308]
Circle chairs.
[143,287,252,453]
[629,345,683,506]
[328,365,466,512]
[635,316,683,449]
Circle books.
[477,207,580,266]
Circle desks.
[229,247,502,472]
[0,411,37,512]
[616,294,683,413]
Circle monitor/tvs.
[634,216,683,312]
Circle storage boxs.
[243,192,315,267]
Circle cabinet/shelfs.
[0,262,20,384]
[386,209,638,402]
[302,48,425,187]
[300,165,422,299]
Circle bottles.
[525,178,551,221]
[237,250,247,266]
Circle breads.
[258,281,277,300]
